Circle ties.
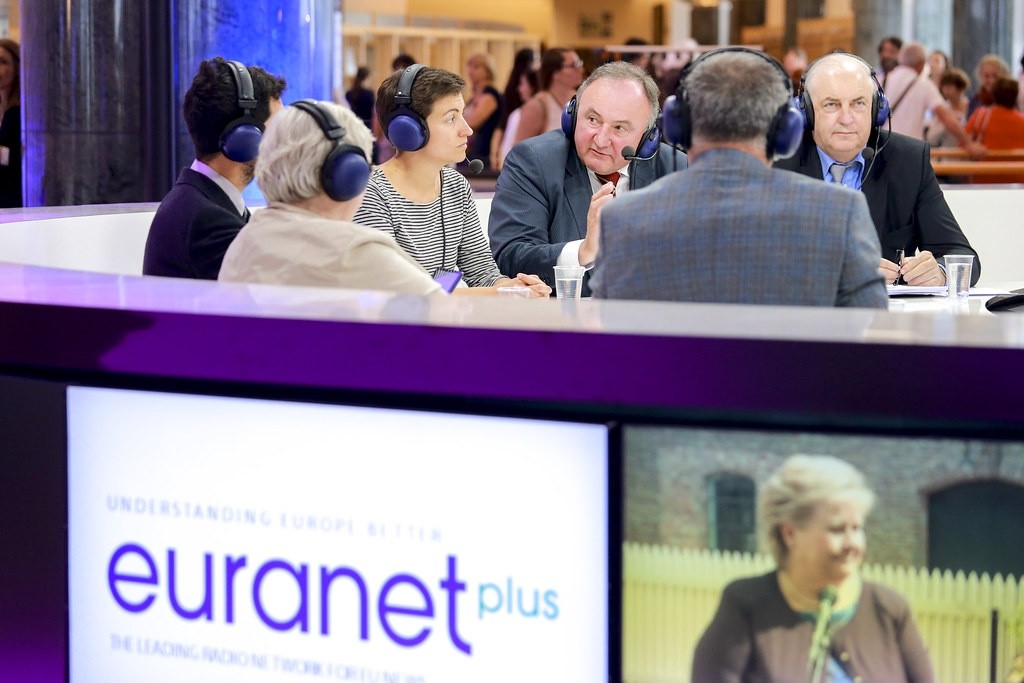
[595,172,620,198]
[830,162,861,184]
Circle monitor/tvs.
[65,370,1024,683]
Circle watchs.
[939,265,947,286]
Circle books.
[888,284,949,296]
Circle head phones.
[798,52,891,127]
[664,46,804,162]
[220,61,267,163]
[561,94,662,158]
[290,99,372,202]
[383,64,431,152]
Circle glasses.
[562,60,584,69]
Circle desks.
[887,283,1024,315]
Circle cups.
[497,287,530,300]
[553,266,585,300]
[943,255,975,300]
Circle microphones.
[621,145,657,160]
[465,157,484,174]
[807,584,837,663]
[862,118,892,161]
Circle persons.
[770,54,981,288]
[352,63,553,299]
[587,46,889,310]
[143,56,285,280]
[218,97,449,296]
[488,60,687,297]
[691,452,935,683]
[0,38,23,207]
[344,35,1024,186]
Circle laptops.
[432,267,464,295]
